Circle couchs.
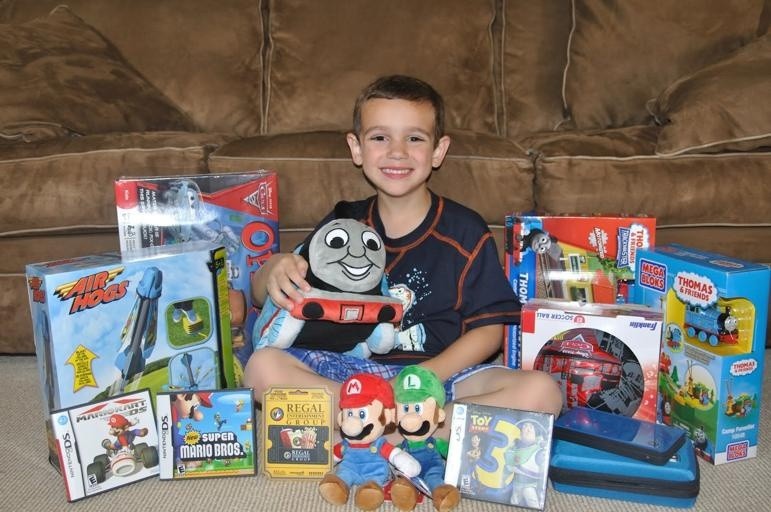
[0,0,771,357]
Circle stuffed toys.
[319,366,461,512]
[255,217,404,358]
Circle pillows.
[0,5,206,143]
[556,0,771,130]
[644,26,771,156]
[262,0,503,141]
[490,0,573,158]
[3,1,265,142]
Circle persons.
[239,73,565,438]
